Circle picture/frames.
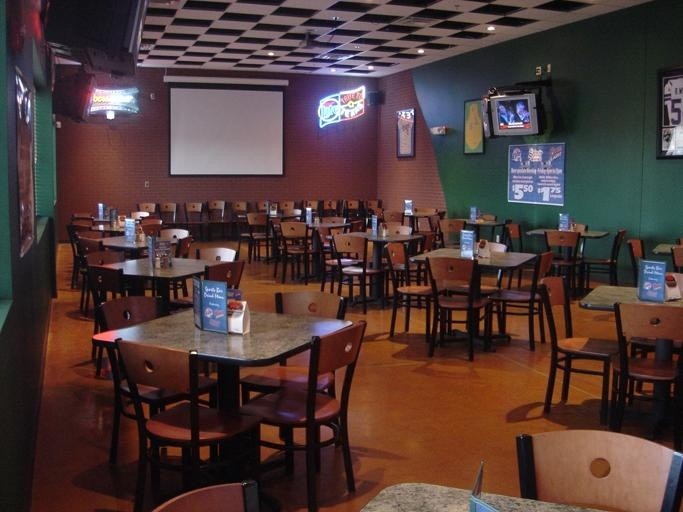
[507,143,564,206]
[463,98,485,154]
[656,68,683,160]
[394,108,418,158]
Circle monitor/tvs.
[53,72,99,121]
[43,0,149,76]
[488,92,545,139]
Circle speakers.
[365,90,384,106]
